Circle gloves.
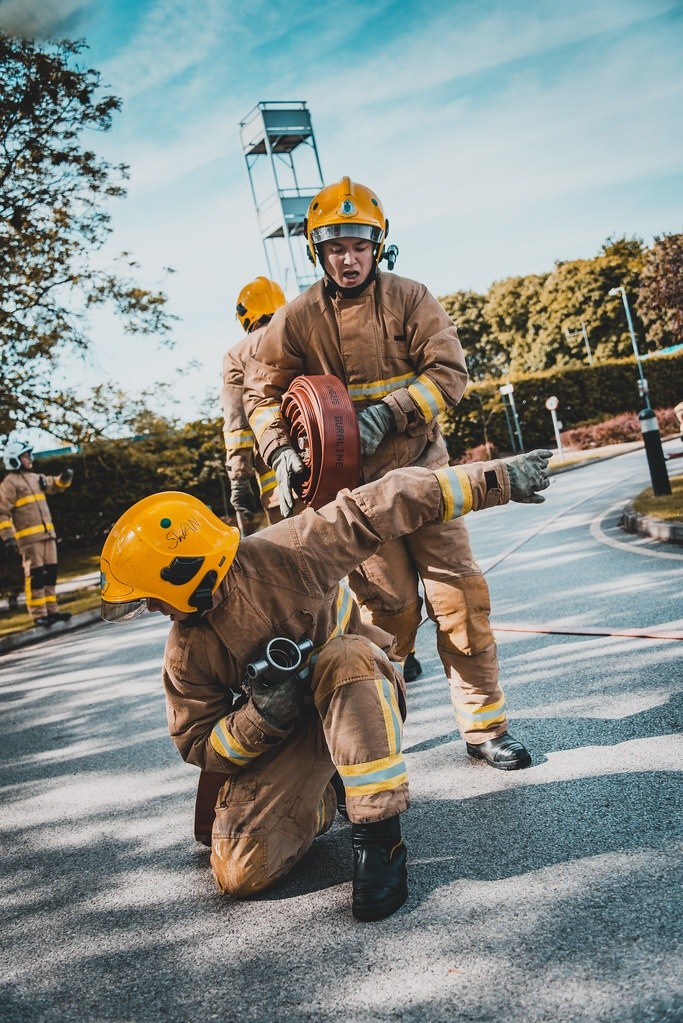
[230,479,258,521]
[505,448,554,504]
[249,673,304,729]
[4,537,18,556]
[270,445,304,519]
[356,403,396,456]
[60,468,73,484]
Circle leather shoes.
[404,651,423,680]
[351,813,407,919]
[466,734,531,770]
[330,770,346,814]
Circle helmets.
[99,490,241,613]
[303,176,389,267]
[236,276,286,335]
[3,442,33,470]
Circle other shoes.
[48,611,72,621]
[33,616,54,626]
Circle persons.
[97,448,550,921]
[241,176,531,770]
[0,438,73,625]
[221,275,290,545]
